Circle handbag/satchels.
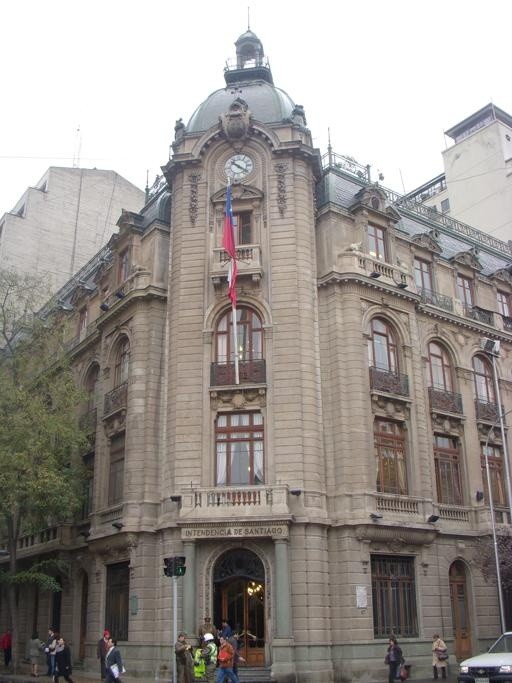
[437,651,448,660]
[384,654,389,664]
[396,666,408,680]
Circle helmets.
[204,633,215,642]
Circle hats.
[103,631,109,636]
[204,617,211,621]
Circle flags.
[223,177,237,308]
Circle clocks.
[223,154,255,180]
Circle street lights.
[480,337,512,518]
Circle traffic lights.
[164,555,186,577]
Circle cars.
[458,632,512,683]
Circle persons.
[42,628,74,683]
[431,634,448,680]
[198,617,246,683]
[27,632,44,677]
[387,636,405,683]
[0,628,12,666]
[97,630,111,680]
[103,637,122,683]
[175,632,194,683]
